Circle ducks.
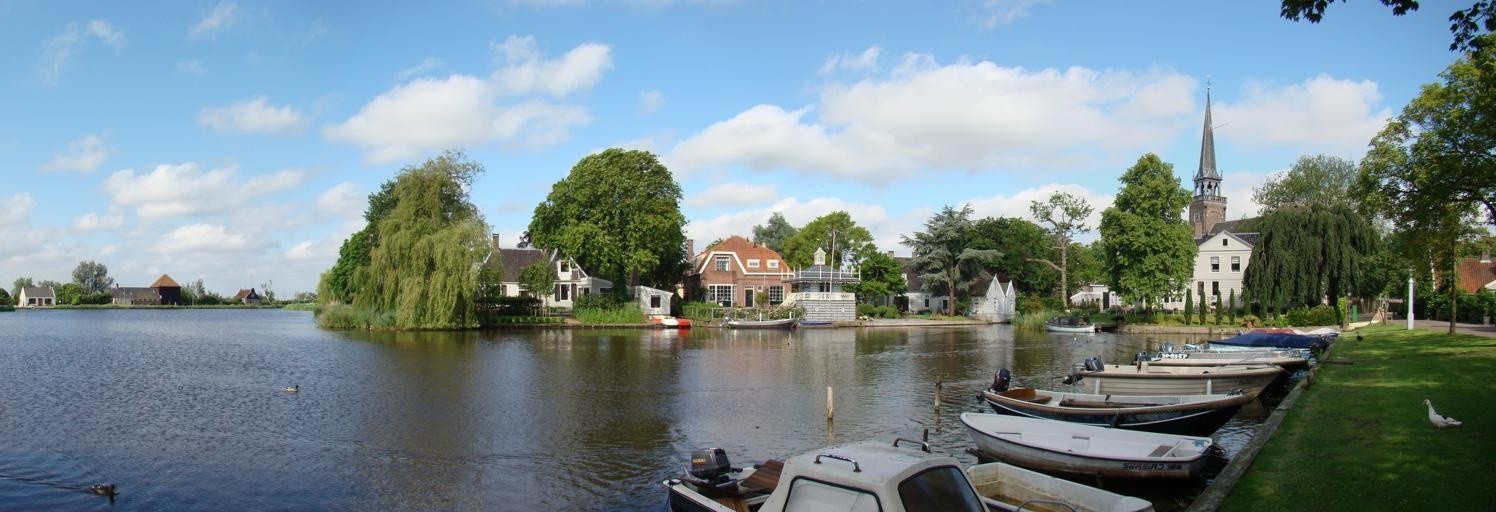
[287,385,299,392]
[1355,331,1364,342]
[90,483,116,496]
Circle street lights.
[1407,266,1416,330]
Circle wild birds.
[1421,398,1463,432]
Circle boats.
[654,314,691,328]
[728,317,796,329]
[1043,316,1095,333]
